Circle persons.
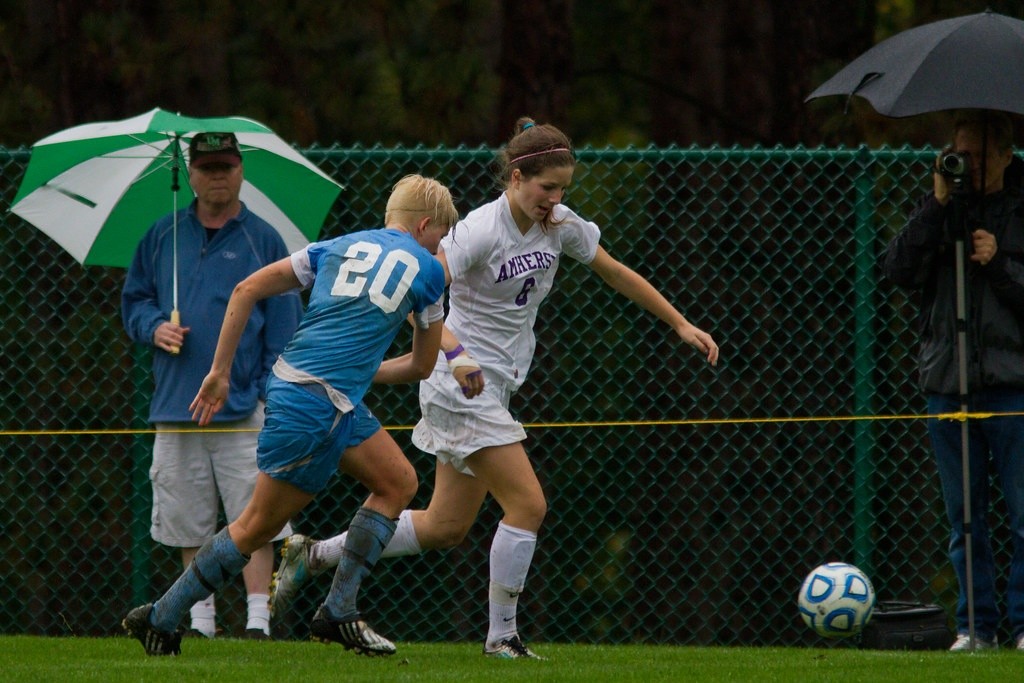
[883,111,1024,650]
[269,118,718,655]
[121,132,302,639]
[122,173,459,655]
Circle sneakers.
[268,534,317,631]
[122,602,186,655]
[483,635,546,661]
[308,603,396,657]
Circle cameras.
[939,151,975,188]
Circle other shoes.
[242,629,272,642]
[185,629,207,639]
[1015,633,1024,649]
[949,635,991,651]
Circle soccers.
[797,562,876,638]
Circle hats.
[190,131,243,168]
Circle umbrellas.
[804,9,1024,233]
[7,106,346,271]
